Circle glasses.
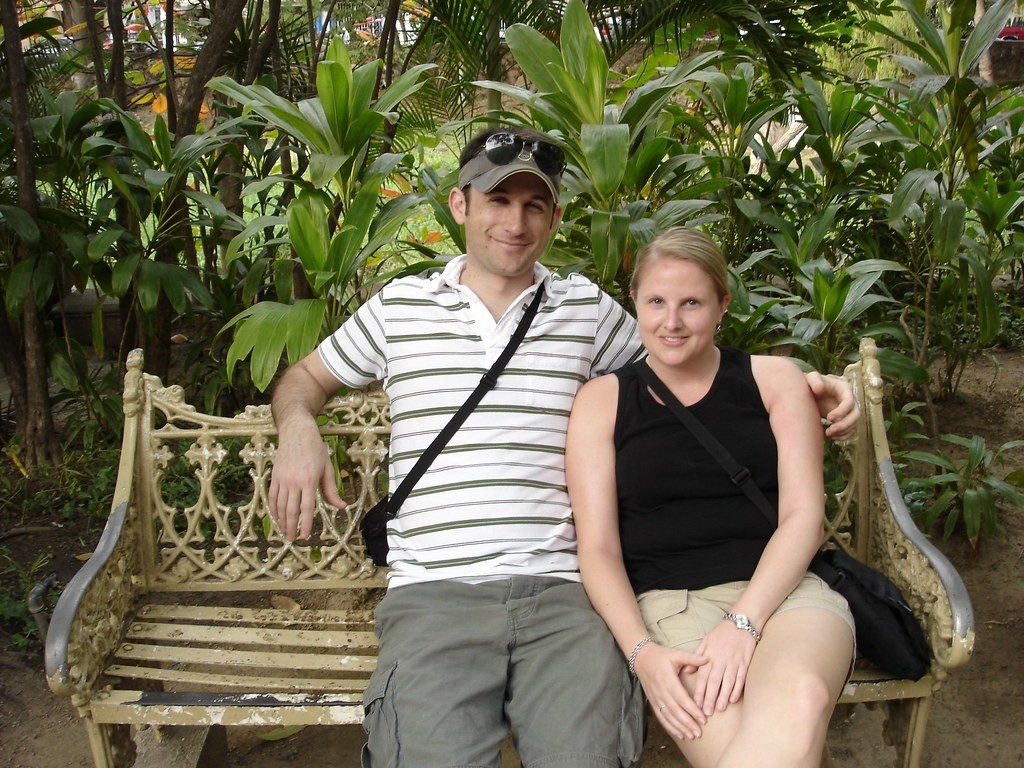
[474,132,567,179]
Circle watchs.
[722,613,761,642]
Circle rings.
[659,705,666,711]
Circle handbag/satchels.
[806,548,932,684]
[359,494,390,567]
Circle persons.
[267,126,861,768]
[565,225,857,768]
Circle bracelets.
[629,637,658,677]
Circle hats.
[458,150,560,205]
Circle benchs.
[43,339,977,768]
